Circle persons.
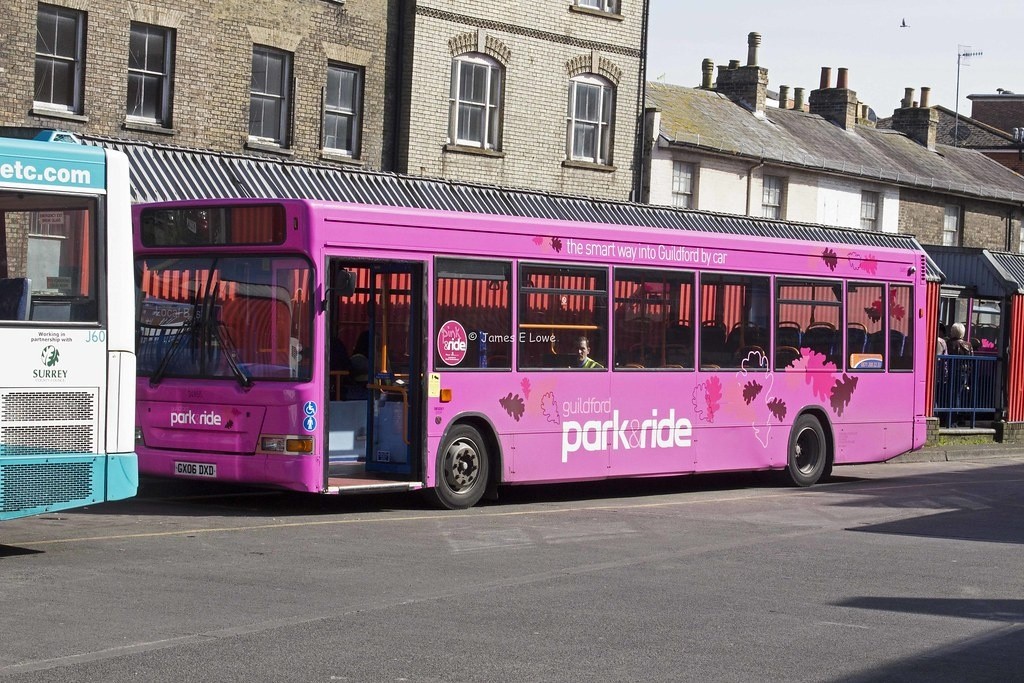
[939,322,972,355]
[351,312,390,367]
[559,335,605,368]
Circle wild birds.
[899,18,911,27]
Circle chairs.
[779,321,802,352]
[776,345,801,368]
[800,322,836,357]
[0,277,32,320]
[628,342,656,367]
[836,322,868,358]
[726,321,759,354]
[352,330,390,360]
[664,344,691,368]
[701,320,726,352]
[659,319,691,349]
[731,345,766,368]
[866,329,905,369]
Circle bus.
[0,137,139,521]
[132,198,927,509]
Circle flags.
[957,43,983,73]
